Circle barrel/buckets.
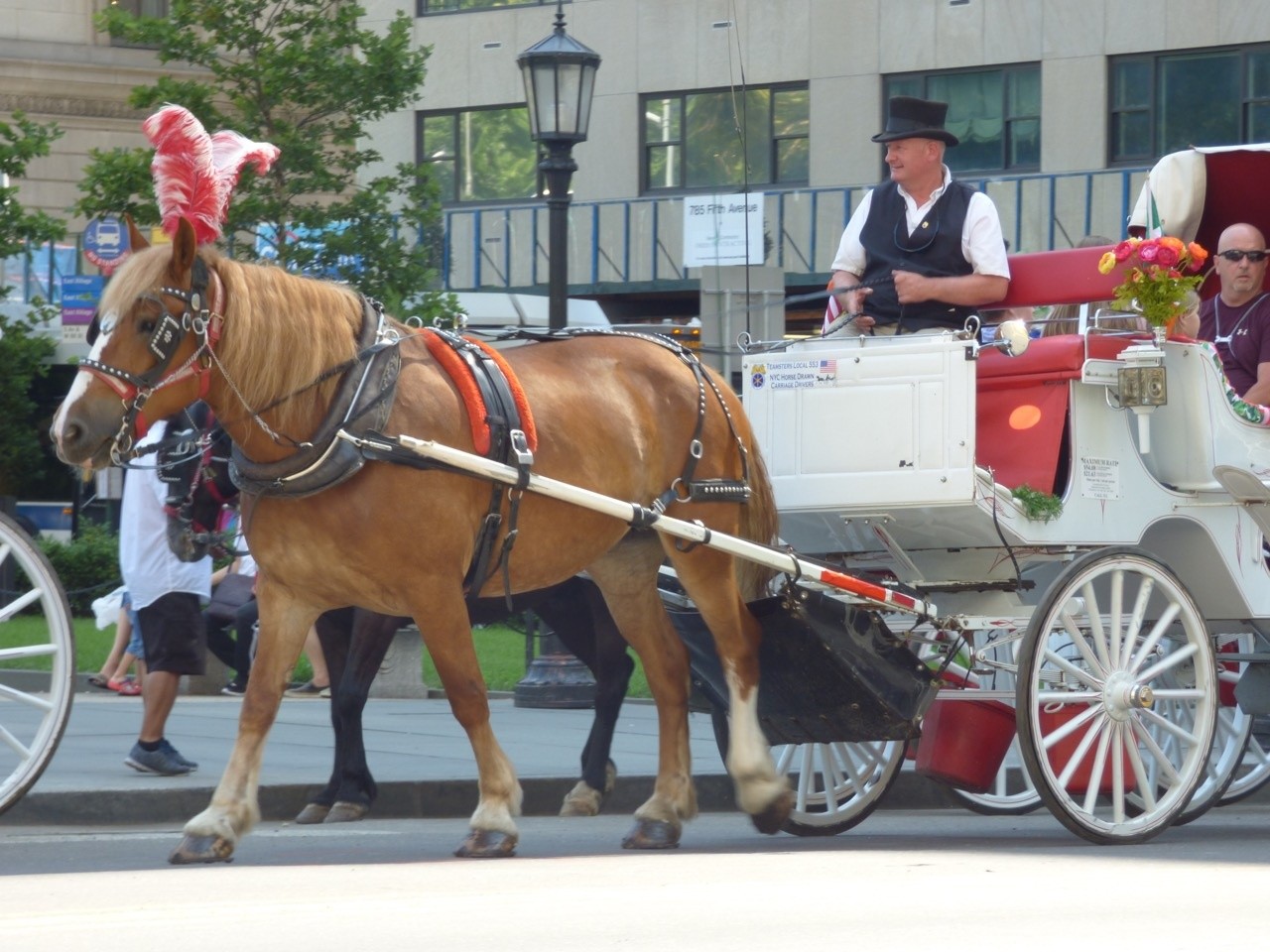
[1211,633,1238,707]
[904,638,1017,795]
[1038,640,1141,792]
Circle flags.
[1146,190,1164,240]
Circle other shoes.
[284,680,332,697]
[87,672,109,689]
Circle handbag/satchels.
[208,573,255,620]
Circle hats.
[871,97,959,148]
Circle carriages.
[49,143,1270,868]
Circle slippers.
[107,676,141,695]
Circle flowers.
[1098,236,1208,324]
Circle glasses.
[1218,249,1268,262]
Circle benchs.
[950,237,1159,500]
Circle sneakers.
[123,742,189,777]
[221,679,246,696]
[161,741,198,771]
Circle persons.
[1198,224,1270,408]
[1174,291,1270,424]
[1041,235,1146,336]
[206,515,330,698]
[826,96,1011,341]
[89,592,144,693]
[981,306,1041,342]
[119,423,213,776]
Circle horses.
[48,214,797,866]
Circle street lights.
[516,0,604,713]
